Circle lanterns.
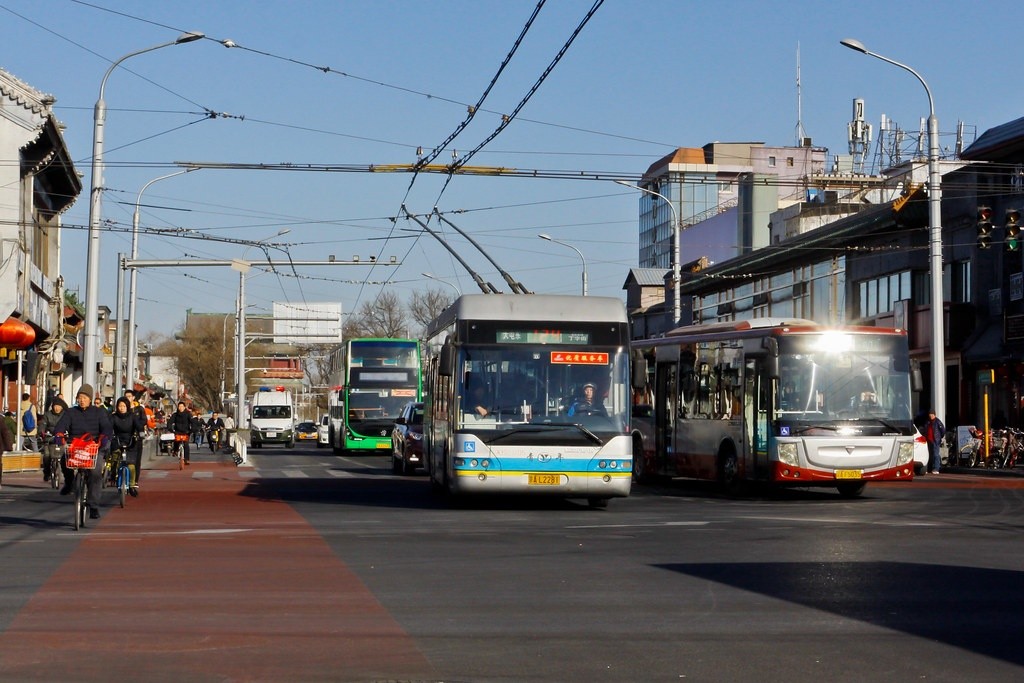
[0,316,36,360]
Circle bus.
[328,338,421,455]
[405,203,634,509]
[630,317,913,498]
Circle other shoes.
[174,450,178,456]
[129,487,136,497]
[61,483,74,495]
[89,507,100,518]
[931,471,939,474]
[185,459,190,465]
[44,472,50,481]
[219,444,222,448]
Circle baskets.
[63,441,99,470]
[175,434,190,441]
[968,437,984,450]
[49,443,64,458]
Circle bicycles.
[172,430,199,471]
[191,424,210,450]
[41,431,142,532]
[960,427,1024,469]
[206,427,226,455]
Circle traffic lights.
[977,207,993,251]
[1005,209,1021,251]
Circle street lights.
[127,167,201,392]
[613,178,681,330]
[82,32,208,406]
[537,233,588,299]
[839,36,946,442]
[420,273,460,297]
[238,229,292,429]
[221,303,255,411]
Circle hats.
[77,384,94,401]
[582,381,598,392]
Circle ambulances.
[246,386,296,449]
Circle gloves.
[101,437,108,447]
[54,436,61,445]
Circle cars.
[913,423,930,476]
[391,401,427,472]
[292,413,330,446]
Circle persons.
[48,394,63,412]
[167,402,196,465]
[145,399,159,437]
[109,397,144,497]
[224,413,234,449]
[94,398,108,411]
[46,384,57,412]
[193,413,208,444]
[48,384,113,519]
[37,398,68,481]
[4,412,17,444]
[567,383,608,417]
[0,411,13,489]
[991,409,1008,427]
[14,393,38,452]
[206,411,225,449]
[124,389,148,489]
[925,409,945,475]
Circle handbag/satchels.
[23,404,35,433]
[68,433,106,467]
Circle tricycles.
[157,428,175,457]
